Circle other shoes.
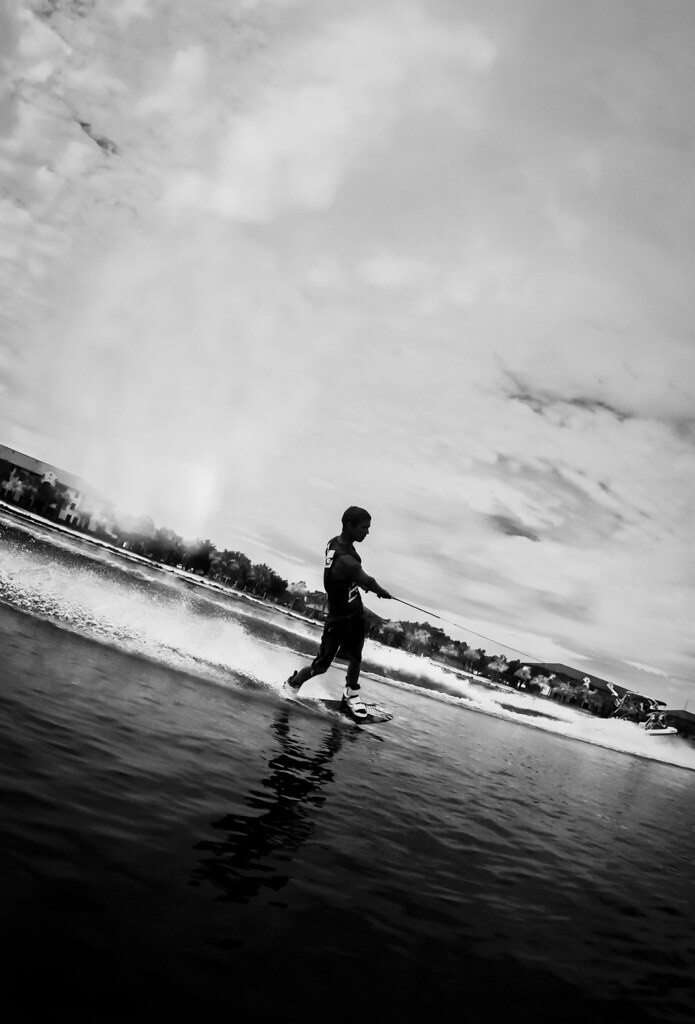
[283,671,300,698]
[343,676,367,718]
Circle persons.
[282,505,391,717]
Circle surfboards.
[162,641,394,725]
